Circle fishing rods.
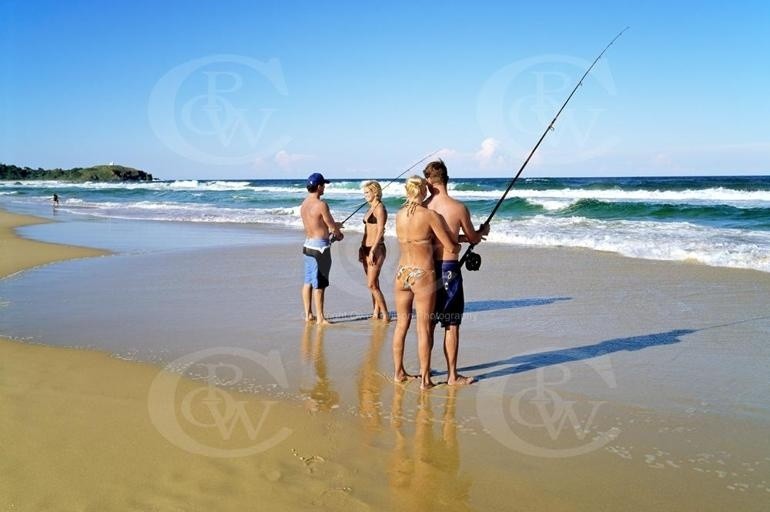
[329,146,446,246]
[458,24,633,271]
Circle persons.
[422,161,490,386]
[393,175,461,390]
[359,181,390,324]
[300,173,343,325]
[54,194,59,204]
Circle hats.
[307,173,331,187]
